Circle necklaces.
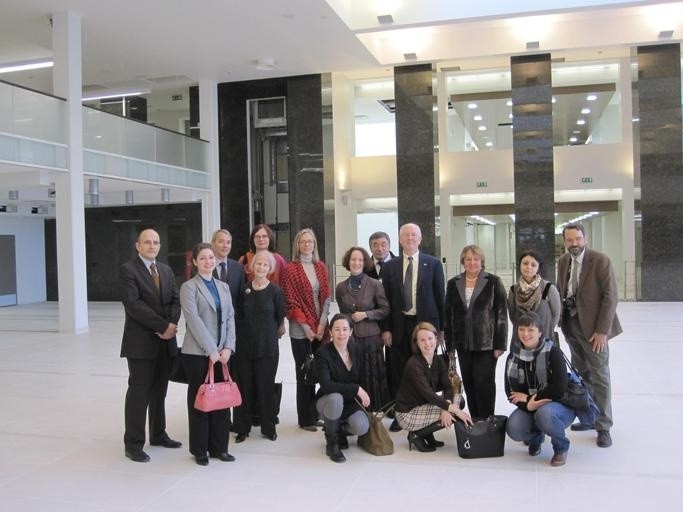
[466,277,477,281]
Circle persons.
[314,224,474,463]
[555,223,622,447]
[503,311,578,466]
[507,251,561,344]
[444,244,507,420]
[119,228,183,462]
[179,223,330,465]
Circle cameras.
[563,296,577,307]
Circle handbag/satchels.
[456,415,506,458]
[357,413,393,457]
[555,370,599,426]
[301,356,315,389]
[193,381,243,412]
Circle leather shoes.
[550,451,566,465]
[149,434,182,448]
[210,453,235,462]
[267,432,277,441]
[124,445,150,463]
[571,423,595,430]
[236,423,251,442]
[196,455,208,467]
[597,430,612,447]
[529,444,541,457]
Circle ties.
[376,262,385,279]
[220,261,228,283]
[570,257,578,297]
[150,262,161,296]
[403,256,413,312]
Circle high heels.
[407,432,445,452]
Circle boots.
[323,417,349,463]
